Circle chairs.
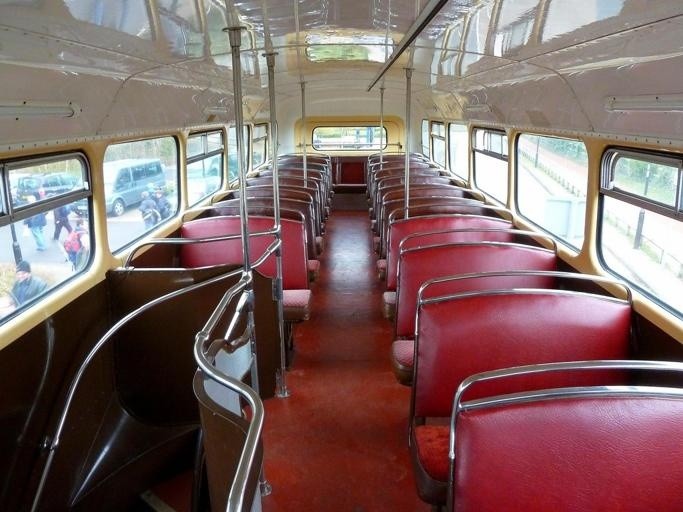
[278,156,334,198]
[446,358,682,510]
[332,155,367,189]
[390,227,558,388]
[377,182,486,280]
[365,151,424,199]
[365,160,430,205]
[207,185,321,283]
[406,269,634,507]
[372,173,467,254]
[279,152,335,189]
[230,174,324,256]
[378,201,515,322]
[267,161,332,206]
[368,168,452,231]
[180,203,313,372]
[256,167,330,221]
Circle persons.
[139,191,161,229]
[62,219,87,271]
[74,233,91,273]
[50,192,73,241]
[39,187,45,199]
[32,191,41,204]
[153,190,173,220]
[7,261,51,309]
[22,195,49,251]
[146,182,158,202]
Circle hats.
[15,260,31,273]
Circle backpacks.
[64,227,87,254]
[142,209,156,226]
[61,204,70,218]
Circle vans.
[69,160,165,216]
[16,172,73,204]
